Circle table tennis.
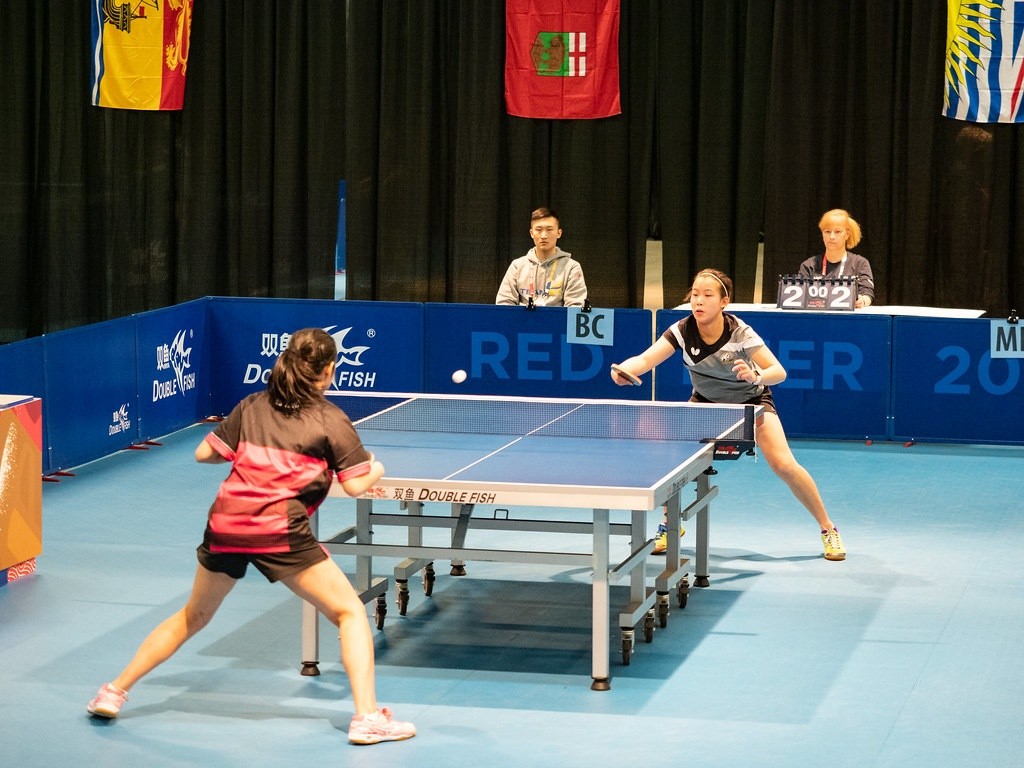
[452,369,467,384]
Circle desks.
[673,302,986,318]
[299,391,764,691]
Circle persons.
[798,209,875,308]
[86,327,417,745]
[611,268,846,561]
[496,208,587,307]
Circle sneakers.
[650,524,685,555]
[86,682,128,718]
[348,705,415,743]
[820,527,846,561]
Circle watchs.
[751,370,762,385]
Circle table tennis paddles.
[610,363,642,387]
[365,451,375,466]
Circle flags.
[90,0,193,110]
[941,0,1024,124]
[504,0,621,120]
